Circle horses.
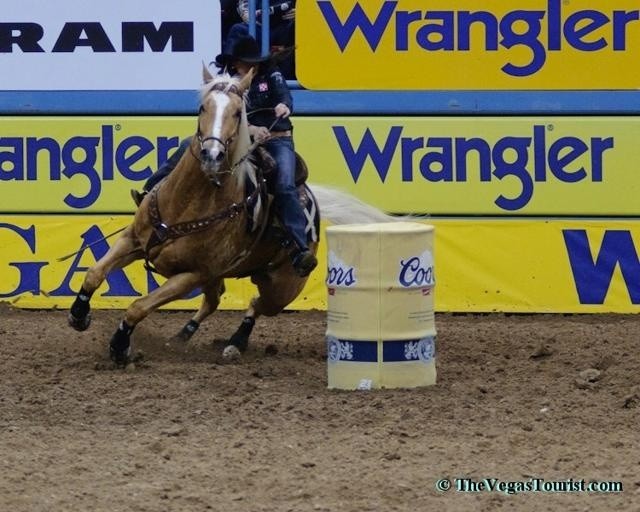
[69,59,434,366]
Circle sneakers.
[131,189,147,205]
[297,254,317,278]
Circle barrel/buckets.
[323,221,439,393]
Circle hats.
[216,35,271,64]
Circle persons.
[132,33,318,277]
[222,0,295,80]
